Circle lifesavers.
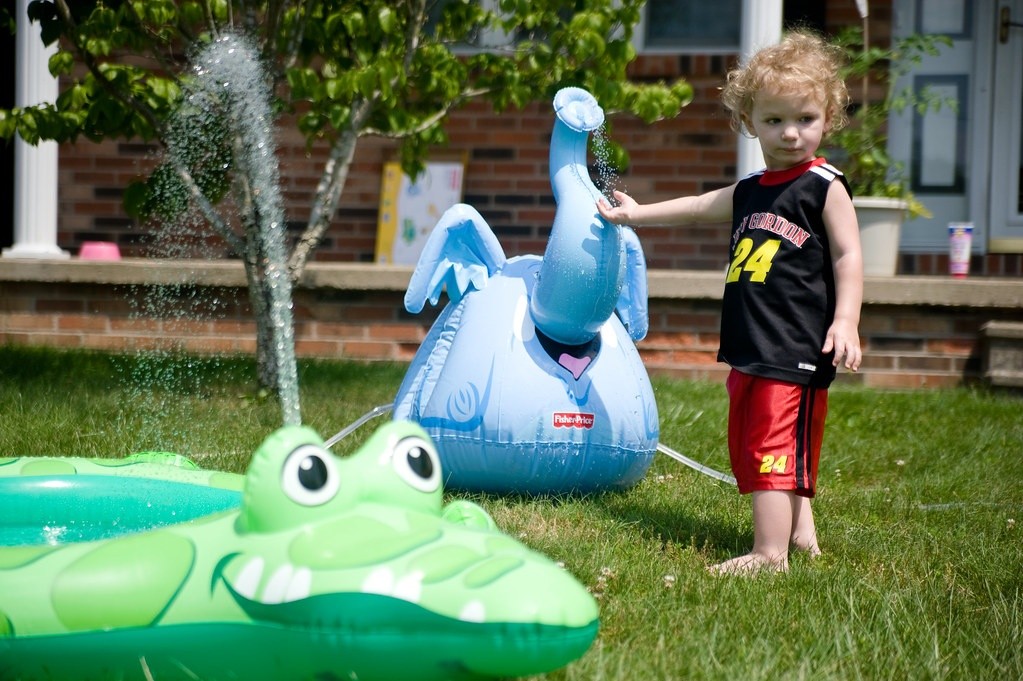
[0,417,601,681]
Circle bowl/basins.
[79,242,121,262]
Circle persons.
[596,28,863,575]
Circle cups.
[948,223,974,279]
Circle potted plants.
[827,23,961,278]
